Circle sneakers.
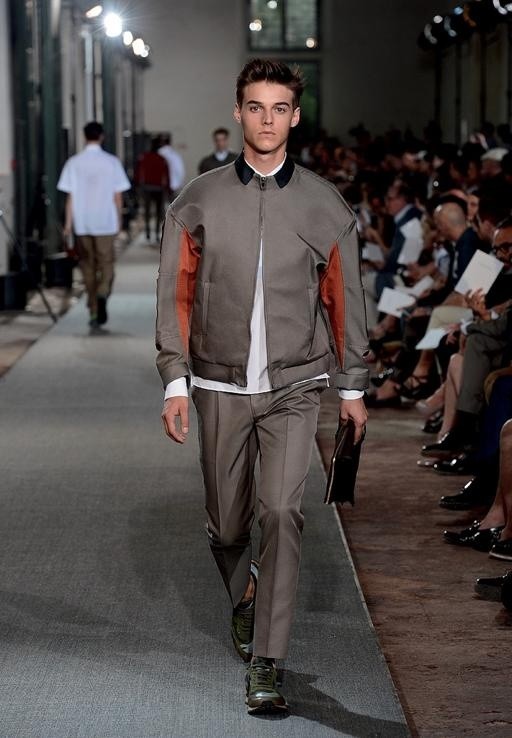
[88,295,109,336]
[244,656,290,716]
[230,559,264,662]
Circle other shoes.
[364,335,442,433]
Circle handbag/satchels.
[323,412,368,507]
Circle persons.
[155,59,369,716]
[157,131,185,221]
[56,122,131,327]
[199,128,238,175]
[135,139,169,241]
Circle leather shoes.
[443,521,511,560]
[419,433,463,457]
[439,476,493,512]
[474,571,512,609]
[430,451,481,472]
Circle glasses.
[492,242,512,254]
